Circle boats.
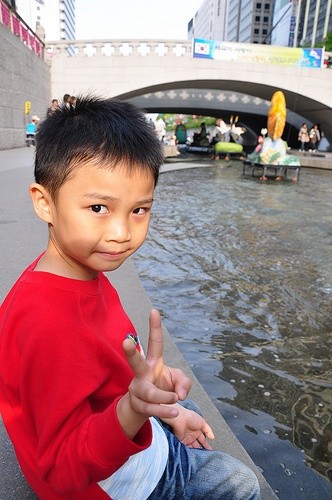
[177,142,246,161]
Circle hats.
[32,115,40,121]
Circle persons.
[298,123,309,152]
[26,94,76,147]
[0,91,260,500]
[309,126,320,153]
[148,114,282,181]
